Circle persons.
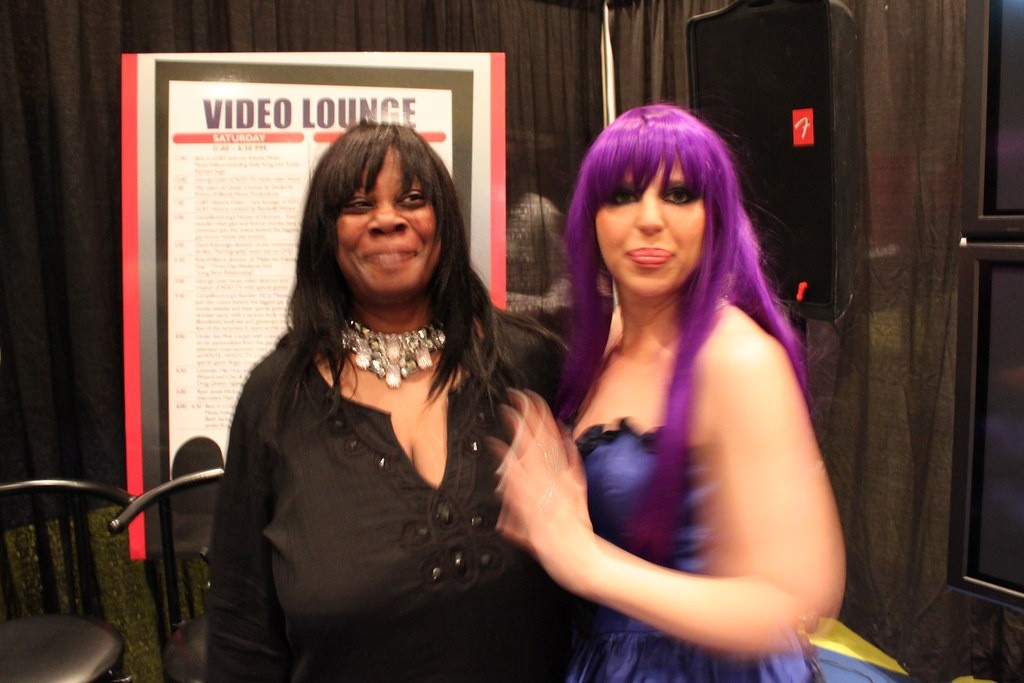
[492,104,916,683]
[505,135,572,414]
[209,123,569,683]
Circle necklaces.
[342,320,446,389]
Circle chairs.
[107,468,225,683]
[0,479,138,683]
